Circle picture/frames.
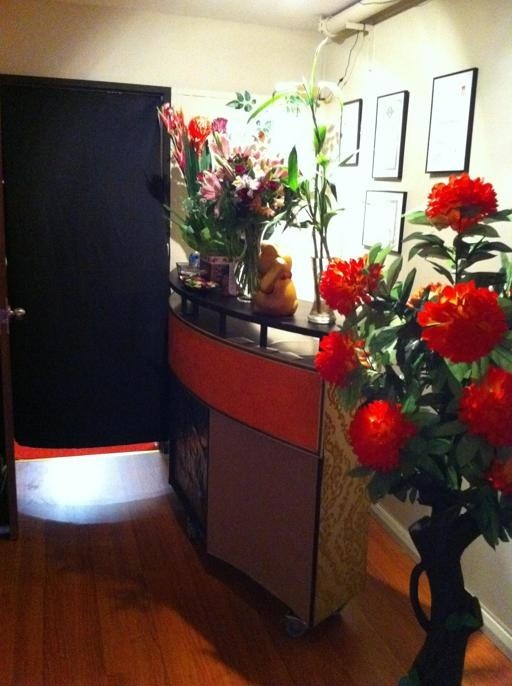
[362,190,407,253]
[340,99,362,166]
[371,90,409,179]
[426,68,478,173]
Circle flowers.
[316,173,512,686]
[155,102,289,256]
[226,35,341,317]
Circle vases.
[199,253,235,296]
[307,223,336,325]
[235,216,276,303]
